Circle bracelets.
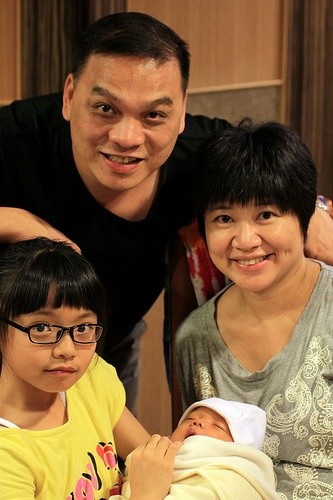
[315,203,328,212]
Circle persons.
[110,397,279,500]
[0,237,183,500]
[0,13,333,476]
[170,118,333,500]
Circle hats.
[177,397,266,450]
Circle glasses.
[0,316,104,344]
